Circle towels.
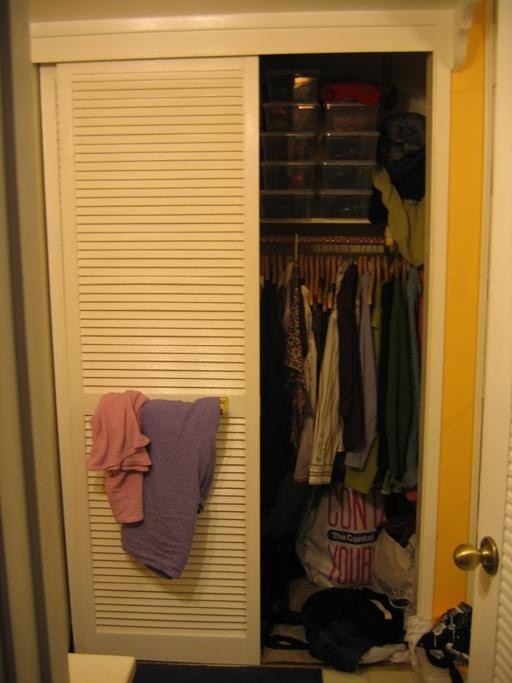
[119,397,219,579]
[86,390,152,525]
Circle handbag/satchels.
[302,585,406,674]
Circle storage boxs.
[261,67,381,219]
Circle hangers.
[260,235,410,307]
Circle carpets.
[131,659,323,683]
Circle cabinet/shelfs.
[29,21,452,667]
